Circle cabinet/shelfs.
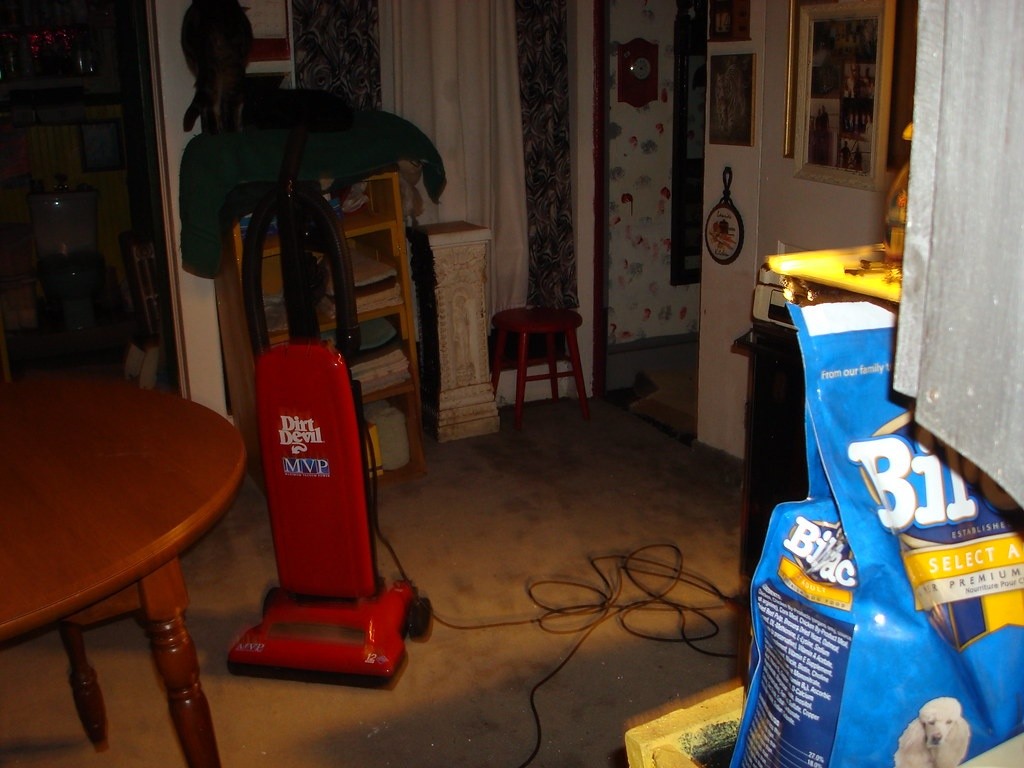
[214,171,427,493]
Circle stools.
[491,303,593,433]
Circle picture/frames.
[779,0,900,193]
[77,116,128,174]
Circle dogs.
[893,697,970,768]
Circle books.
[313,241,411,398]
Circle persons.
[813,15,877,174]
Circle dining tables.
[0,377,251,768]
